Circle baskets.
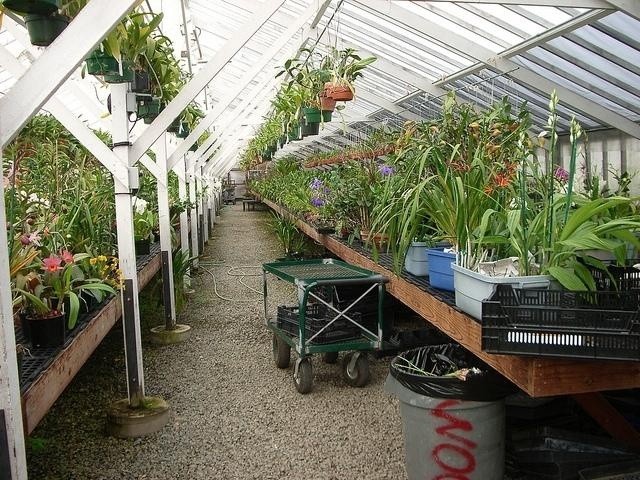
[277,301,363,345]
[481,255,640,364]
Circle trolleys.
[262,257,389,393]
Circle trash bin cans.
[384,343,506,480]
